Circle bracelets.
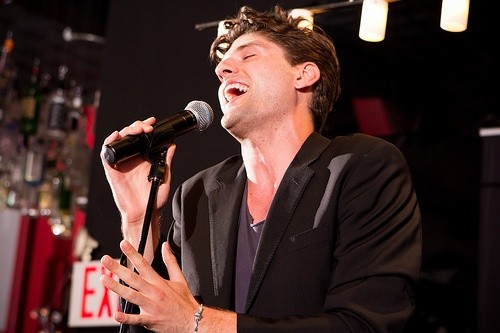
[188,302,208,333]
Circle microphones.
[102,99,215,165]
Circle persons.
[93,3,428,333]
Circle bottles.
[0,33,103,239]
